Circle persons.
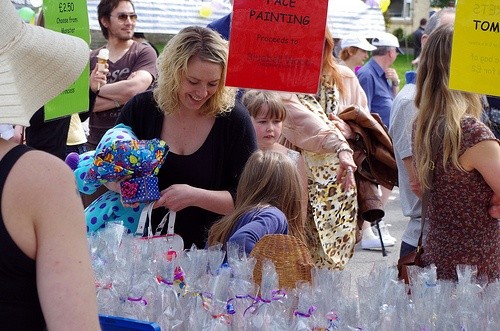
[389,0,500,297]
[0,0,103,331]
[14,33,160,163]
[26,0,406,270]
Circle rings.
[347,169,353,173]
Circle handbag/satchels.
[397,249,421,295]
[133,202,184,259]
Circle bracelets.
[355,133,359,143]
[336,147,354,159]
[114,100,121,108]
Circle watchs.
[392,80,400,86]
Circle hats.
[341,34,377,51]
[1,0,90,127]
[370,33,405,56]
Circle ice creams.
[96,48,109,64]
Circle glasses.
[109,14,138,24]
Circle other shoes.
[372,223,396,243]
[361,227,394,248]
